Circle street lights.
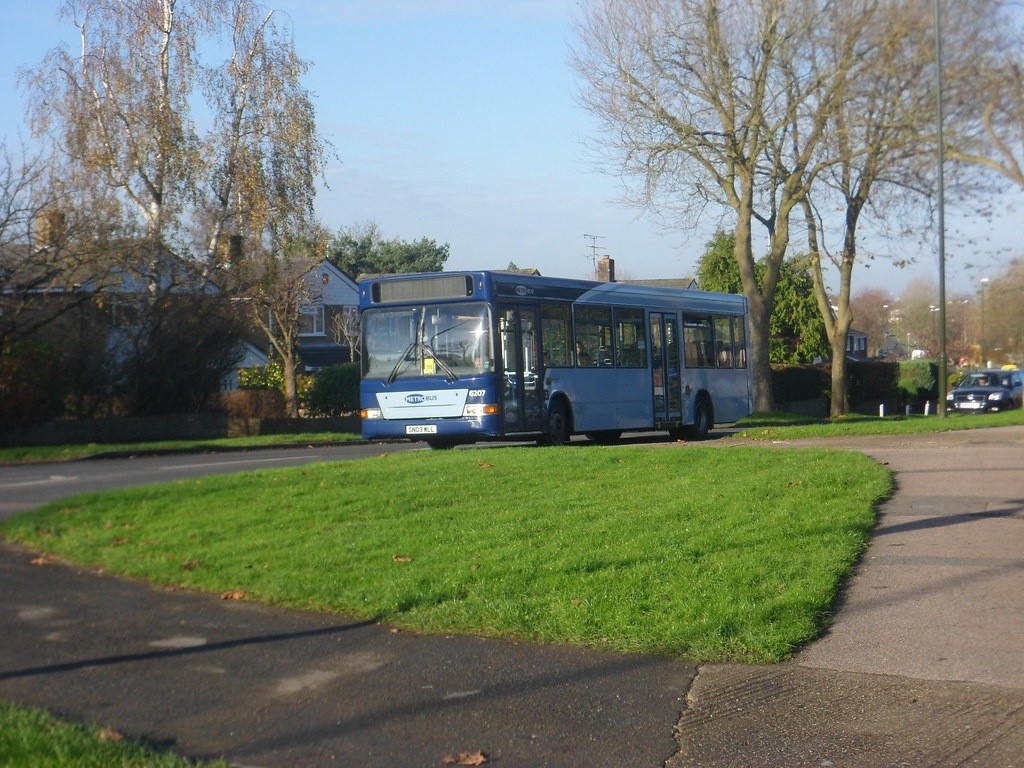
[881,277,991,368]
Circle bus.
[353,270,756,451]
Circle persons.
[571,341,591,366]
[977,378,988,386]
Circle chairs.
[597,345,638,368]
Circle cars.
[945,369,1024,413]
